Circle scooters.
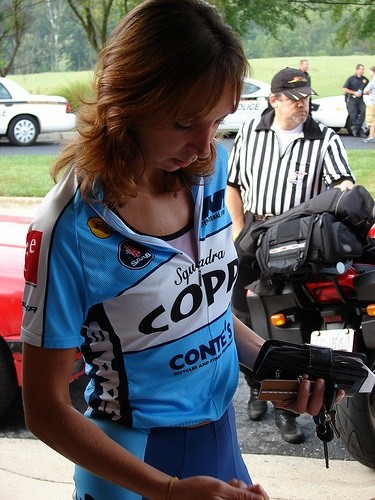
[301,240,374,474]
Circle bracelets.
[167,477,178,500]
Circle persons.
[298,60,309,75]
[20,0,344,500]
[225,67,356,443]
[342,64,370,137]
[362,66,375,143]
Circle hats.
[271,67,319,101]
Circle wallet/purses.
[254,339,369,398]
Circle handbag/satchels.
[231,185,375,314]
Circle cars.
[215,77,277,139]
[309,96,371,136]
[0,76,76,147]
[0,208,90,411]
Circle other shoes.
[362,137,375,143]
[351,126,357,137]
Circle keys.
[317,424,334,469]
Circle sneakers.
[247,388,268,421]
[275,413,305,444]
